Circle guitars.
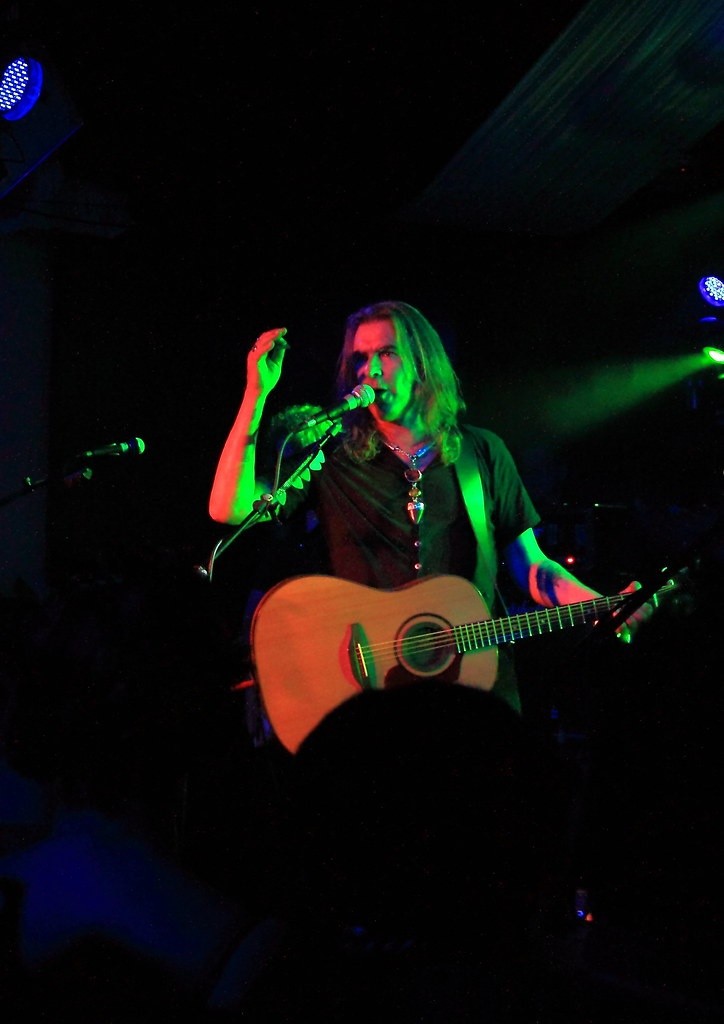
[248,566,701,758]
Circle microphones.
[296,383,377,431]
[81,435,146,459]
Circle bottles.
[575,872,589,920]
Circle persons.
[207,302,653,713]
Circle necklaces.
[381,436,438,528]
